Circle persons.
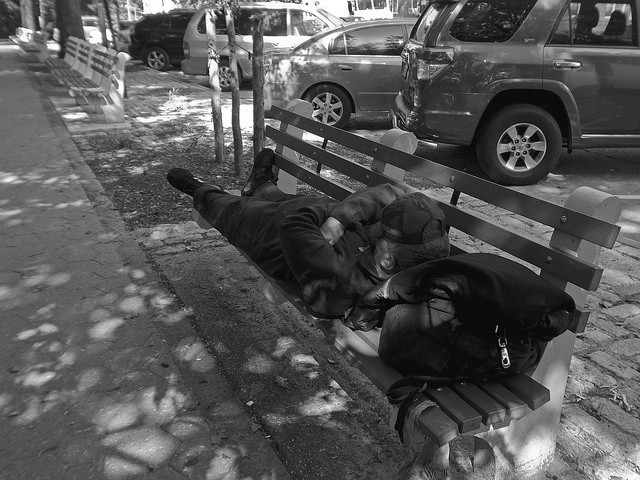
[166,148,452,317]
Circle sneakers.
[167,168,224,197]
[241,149,279,196]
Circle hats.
[369,192,446,245]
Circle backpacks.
[378,297,547,444]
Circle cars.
[263,17,418,128]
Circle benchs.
[8,26,49,63]
[189,97,621,479]
[44,36,132,123]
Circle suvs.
[391,0,639,185]
[180,4,348,90]
[128,8,198,70]
